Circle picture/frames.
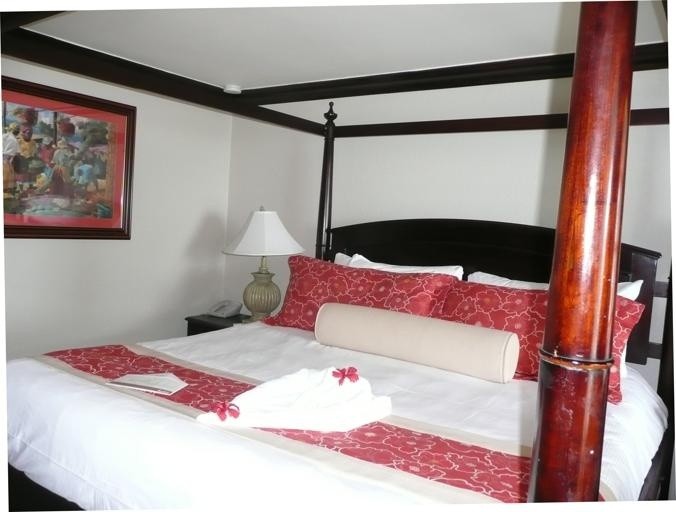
[1,75,137,240]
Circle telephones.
[208,299,243,318]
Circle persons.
[2,120,109,219]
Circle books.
[104,370,189,397]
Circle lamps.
[221,204,306,323]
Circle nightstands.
[184,313,251,337]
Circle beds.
[5,219,668,512]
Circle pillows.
[313,302,520,383]
[334,253,465,282]
[262,253,461,332]
[431,280,646,405]
[467,271,646,378]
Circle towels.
[196,366,395,434]
